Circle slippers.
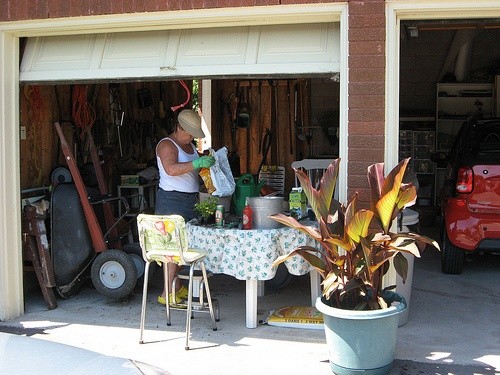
[176,284,188,300]
[158,293,187,309]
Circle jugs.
[231,174,265,217]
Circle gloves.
[192,155,215,169]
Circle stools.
[136,213,220,351]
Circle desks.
[186,216,319,329]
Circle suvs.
[431,113,500,275]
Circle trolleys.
[47,122,158,300]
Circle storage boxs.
[120,175,139,185]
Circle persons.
[154,110,215,308]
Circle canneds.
[215,205,224,227]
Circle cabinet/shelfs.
[118,183,160,244]
[398,74,500,209]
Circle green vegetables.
[192,197,218,217]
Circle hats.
[178,109,205,138]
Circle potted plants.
[268,156,441,375]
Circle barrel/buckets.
[246,196,284,230]
[199,192,231,219]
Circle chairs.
[290,158,339,201]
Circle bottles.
[289,187,307,221]
[242,197,252,230]
